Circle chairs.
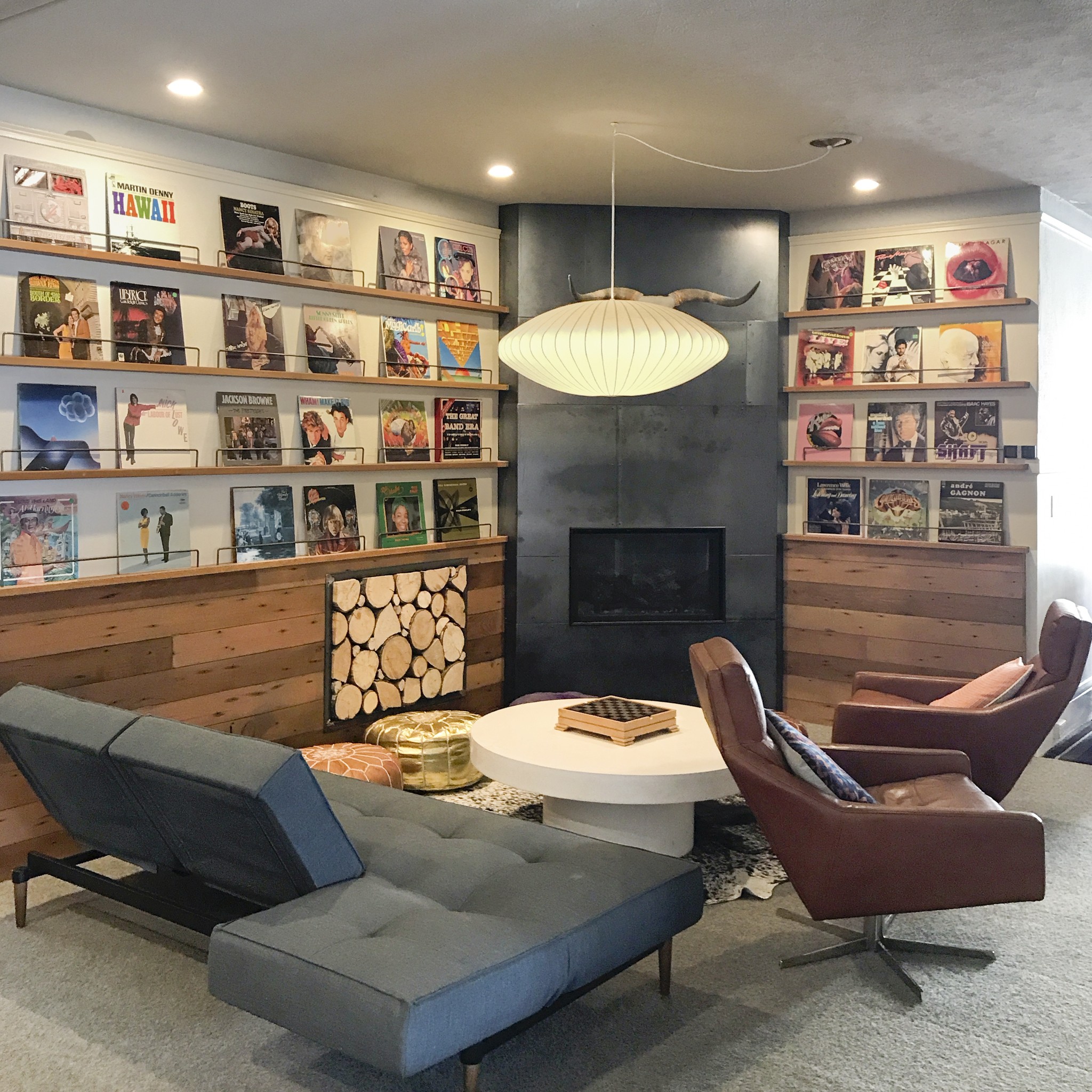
[687,638,1050,1004]
[830,600,1092,803]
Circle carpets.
[416,774,794,908]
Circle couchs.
[2,680,708,1090]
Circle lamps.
[494,116,733,399]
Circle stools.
[361,710,485,791]
[297,742,403,790]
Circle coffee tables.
[469,698,743,859]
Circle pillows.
[926,656,1035,709]
[765,708,876,805]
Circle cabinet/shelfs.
[1,234,514,601]
[780,299,1031,557]
